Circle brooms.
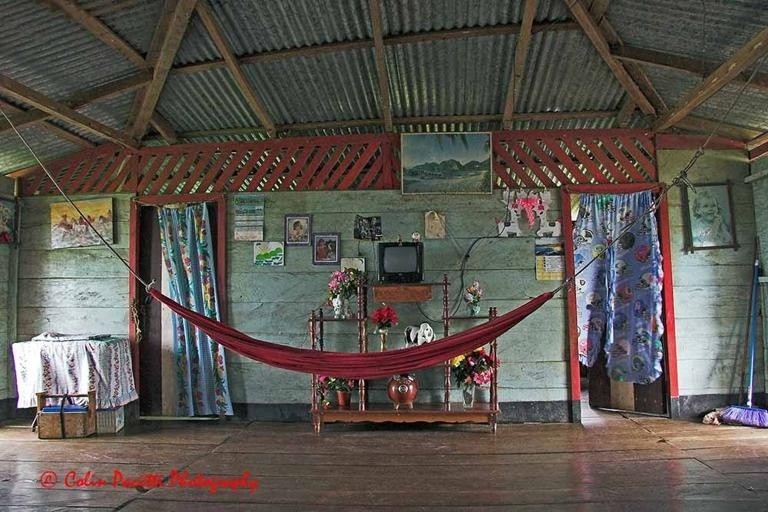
[717,262,768,430]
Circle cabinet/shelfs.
[306,275,499,433]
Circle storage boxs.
[34,389,97,440]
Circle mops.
[703,237,757,426]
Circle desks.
[13,337,133,433]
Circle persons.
[690,188,731,244]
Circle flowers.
[369,300,399,329]
[450,345,500,387]
[326,266,370,301]
[464,280,485,305]
[315,374,354,409]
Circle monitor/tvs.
[378,242,424,284]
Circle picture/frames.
[312,232,341,266]
[681,182,740,253]
[284,214,314,245]
[400,132,493,195]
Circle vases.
[462,381,474,411]
[334,388,351,408]
[331,292,352,320]
[376,326,388,352]
[471,302,481,316]
